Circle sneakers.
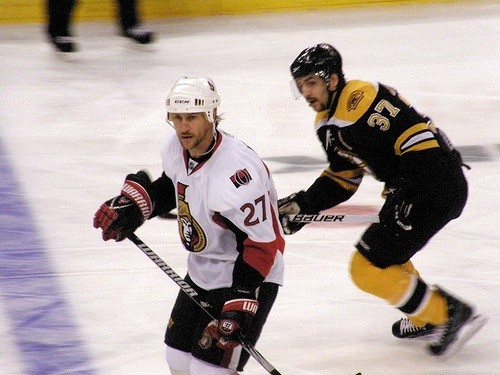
[428,284,488,361]
[391,318,436,340]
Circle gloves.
[276,190,318,235]
[378,201,417,235]
[93,172,155,243]
[204,293,260,351]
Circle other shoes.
[127,27,152,44]
[50,34,78,53]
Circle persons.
[278,44,488,362]
[93,75,285,374]
[42,0,158,54]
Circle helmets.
[292,43,342,86]
[165,75,218,122]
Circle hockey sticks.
[157,211,396,225]
[125,230,362,375]
